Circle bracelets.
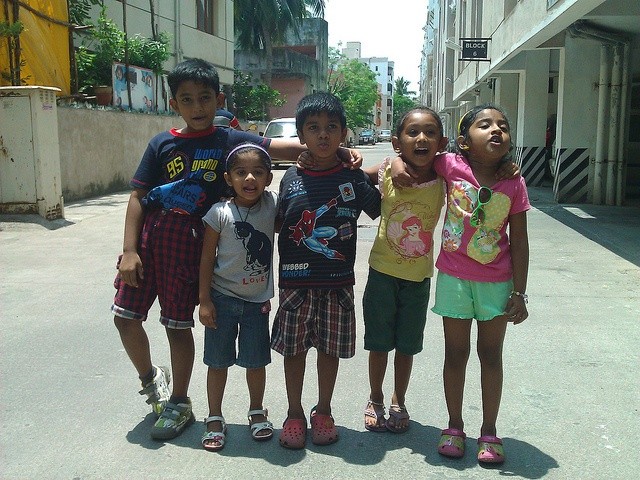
[510,290,528,303]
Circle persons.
[197,141,281,452]
[212,108,244,133]
[221,92,382,448]
[112,64,363,440]
[296,108,520,433]
[390,106,531,463]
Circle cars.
[259,116,308,166]
[375,130,378,143]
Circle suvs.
[358,129,375,145]
[378,130,391,142]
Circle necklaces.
[233,198,251,231]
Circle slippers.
[438,428,466,457]
[478,436,505,463]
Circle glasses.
[468,185,493,227]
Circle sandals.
[201,415,228,451]
[310,406,338,445]
[386,405,410,433]
[364,400,388,432]
[279,417,307,449]
[247,408,273,441]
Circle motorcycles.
[213,116,258,133]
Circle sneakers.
[150,395,195,440]
[139,365,171,419]
